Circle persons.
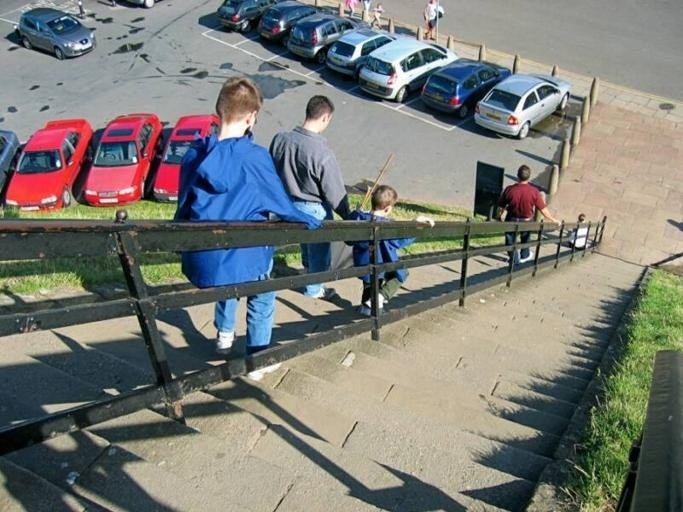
[496,165,561,265]
[371,4,384,29]
[568,214,588,250]
[424,0,438,40]
[359,0,371,21]
[269,96,352,300]
[344,186,435,317]
[346,0,359,17]
[174,76,327,382]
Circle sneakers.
[248,362,281,381]
[313,288,335,300]
[211,340,234,362]
[361,298,385,316]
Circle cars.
[5,119,91,215]
[421,60,507,117]
[84,113,164,206]
[154,115,219,203]
[472,72,571,139]
[119,0,156,12]
[17,9,97,58]
[0,129,20,203]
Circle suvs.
[286,13,366,61]
[215,0,282,30]
[358,37,461,105]
[257,1,318,47]
[327,26,403,78]
